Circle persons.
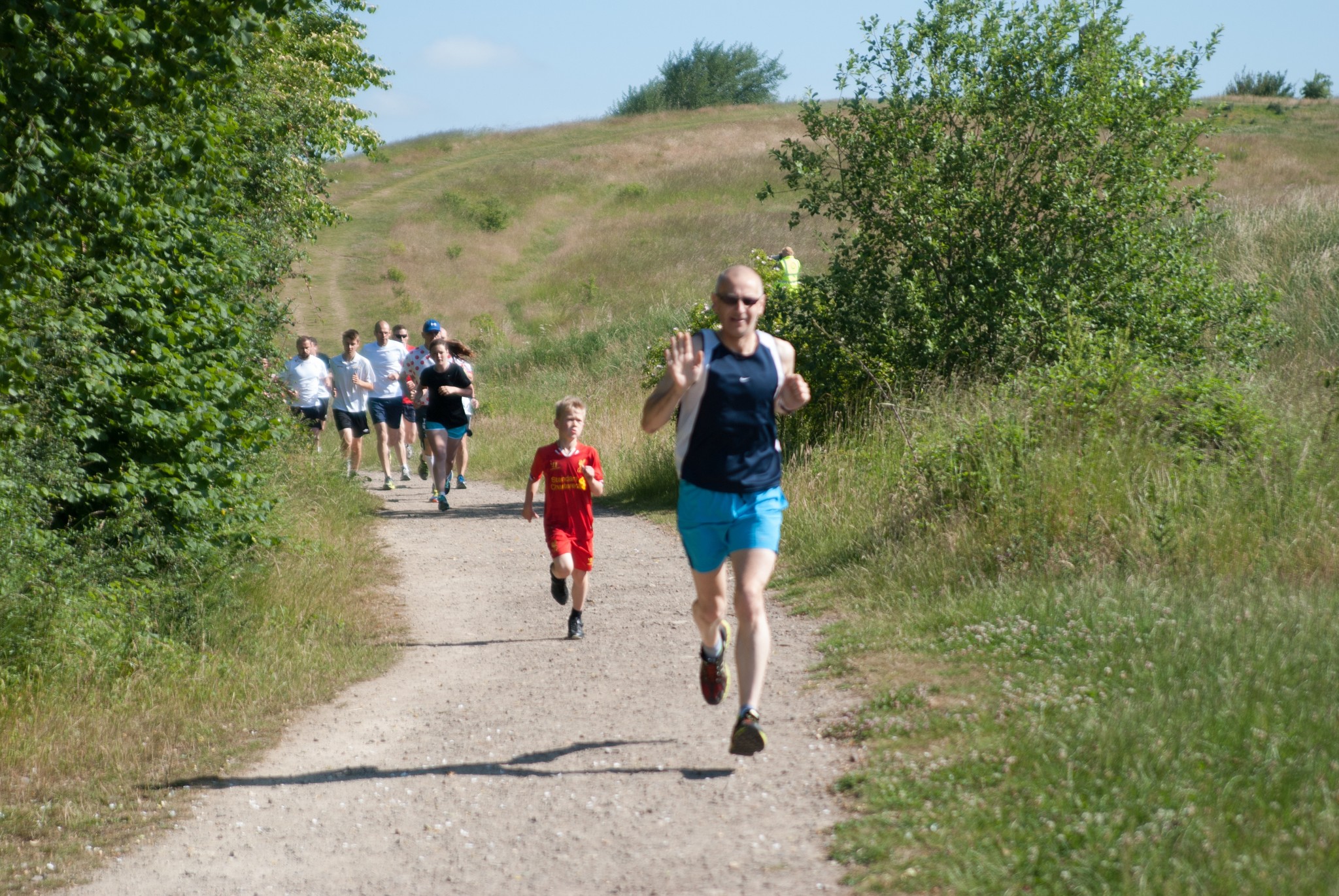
[774,247,801,291]
[636,265,811,761]
[522,396,604,642]
[258,320,479,512]
[280,335,329,454]
[326,328,376,476]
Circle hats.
[423,319,440,332]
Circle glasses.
[717,293,761,305]
[394,334,407,338]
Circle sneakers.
[567,618,584,639]
[699,620,731,705]
[405,444,413,459]
[400,467,411,480]
[456,476,467,488]
[438,495,450,511]
[729,710,763,755]
[418,454,429,480]
[383,477,395,489]
[429,490,438,502]
[445,473,451,495]
[550,562,569,605]
